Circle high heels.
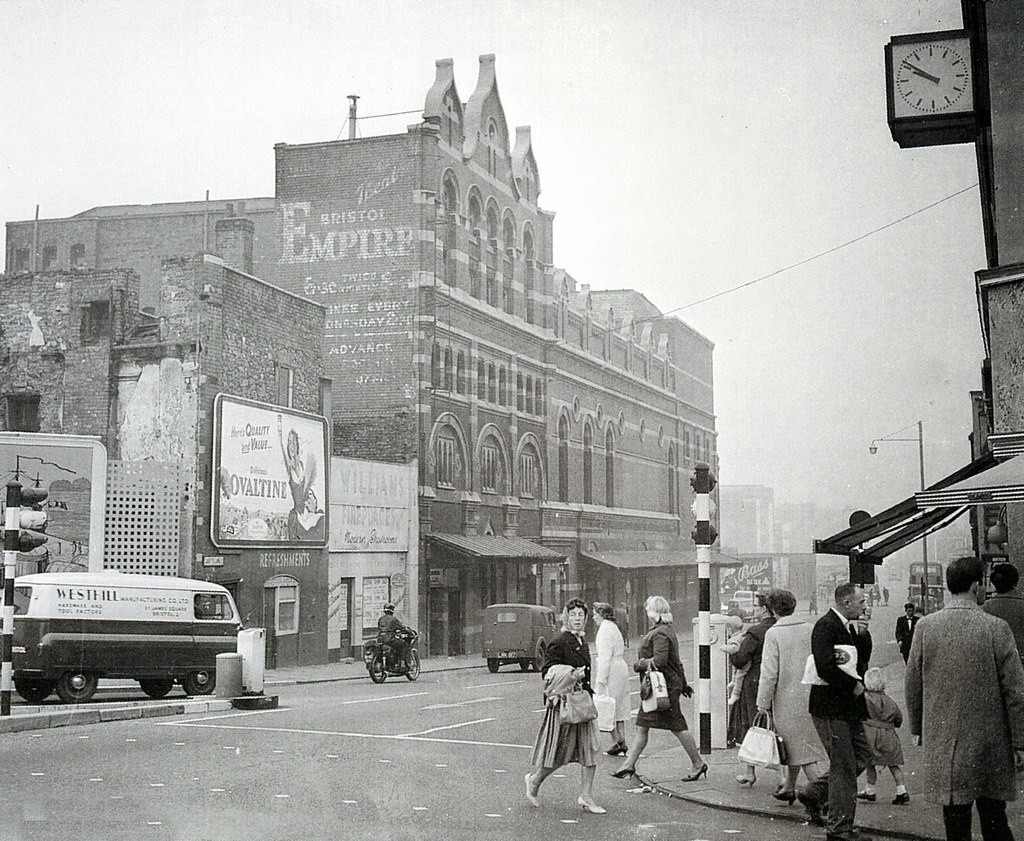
[524,773,539,807]
[607,740,628,756]
[681,763,708,782]
[609,767,635,780]
[577,796,607,814]
[774,791,796,805]
[736,774,756,788]
[776,778,786,791]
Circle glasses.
[569,600,584,609]
[753,603,760,606]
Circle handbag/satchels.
[640,658,670,712]
[738,711,788,771]
[560,671,598,724]
[801,643,862,686]
[592,683,615,732]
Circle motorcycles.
[360,624,420,685]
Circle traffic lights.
[19,487,49,553]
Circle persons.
[376,603,415,671]
[903,557,1024,841]
[525,596,606,814]
[592,567,927,841]
[980,564,1024,670]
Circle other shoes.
[857,791,876,801]
[798,790,824,826]
[892,792,910,805]
[826,831,872,841]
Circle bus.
[906,561,945,614]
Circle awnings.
[580,549,745,571]
[424,532,566,564]
[915,451,1024,508]
[811,450,1001,566]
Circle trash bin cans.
[237,627,267,696]
[216,653,242,698]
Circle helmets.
[382,603,395,613]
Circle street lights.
[869,420,929,617]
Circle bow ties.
[907,618,911,620]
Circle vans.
[3,572,247,706]
[733,590,767,622]
[481,602,563,673]
